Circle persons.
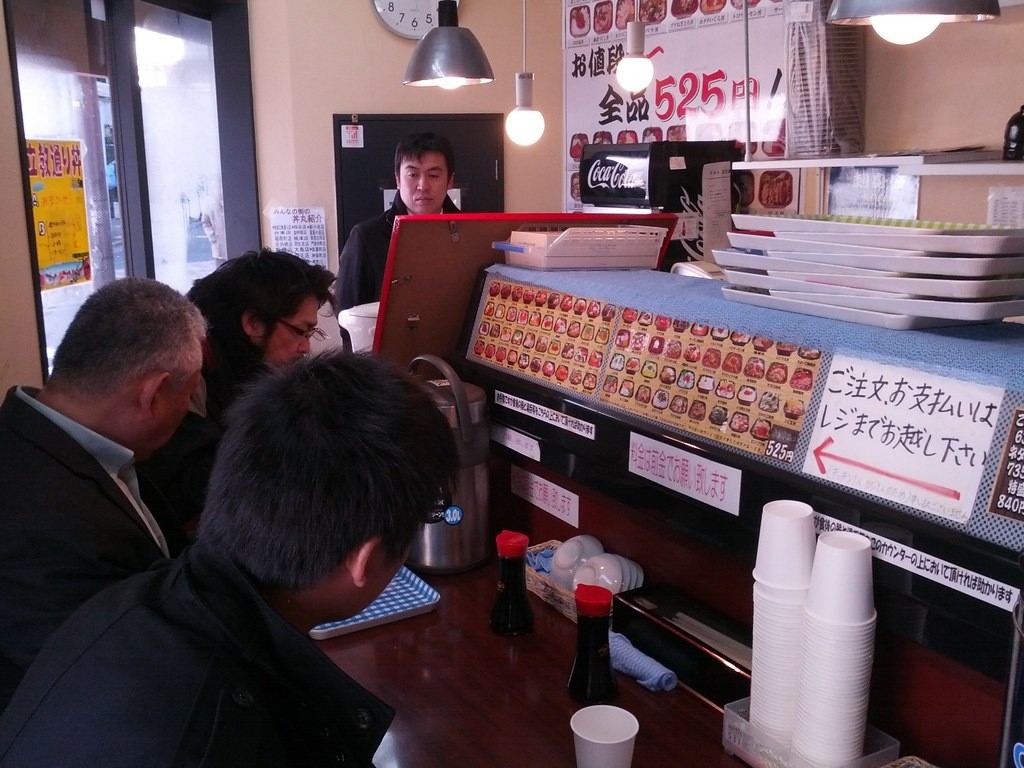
[0,277,208,713]
[135,246,339,559]
[104,124,117,218]
[333,131,462,340]
[0,351,459,768]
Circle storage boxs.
[721,697,902,768]
[493,224,669,270]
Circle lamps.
[616,0,653,92]
[826,1,1001,43]
[504,0,545,145]
[401,0,494,91]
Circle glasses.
[278,318,329,341]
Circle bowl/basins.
[758,171,793,207]
[731,0,759,10]
[667,125,686,142]
[639,0,667,25]
[593,131,613,145]
[569,134,589,162]
[617,130,637,144]
[730,122,757,156]
[670,0,698,19]
[551,534,644,595]
[474,280,820,441]
[570,6,591,38]
[594,1,613,33]
[762,119,787,156]
[700,0,727,15]
[642,127,662,142]
[733,171,754,206]
[617,0,635,29]
[571,172,580,200]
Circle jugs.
[403,353,488,576]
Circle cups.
[570,705,640,768]
[749,500,879,760]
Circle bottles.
[569,586,619,704]
[492,530,532,634]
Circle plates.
[789,22,865,155]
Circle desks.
[312,561,757,768]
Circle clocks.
[369,0,462,41]
